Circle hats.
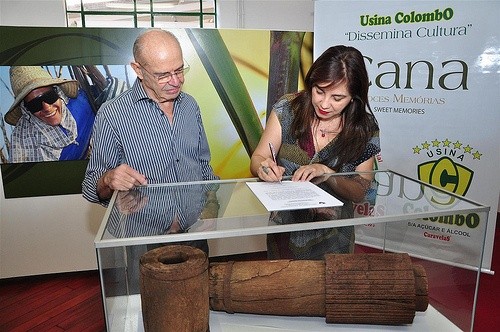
[4,66,79,126]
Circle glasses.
[24,87,59,114]
[138,58,190,83]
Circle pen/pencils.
[268,142,282,184]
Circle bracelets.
[207,199,220,209]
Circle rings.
[262,166,270,174]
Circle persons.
[4,66,128,163]
[250,45,381,261]
[82,28,222,257]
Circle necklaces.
[315,117,343,137]
